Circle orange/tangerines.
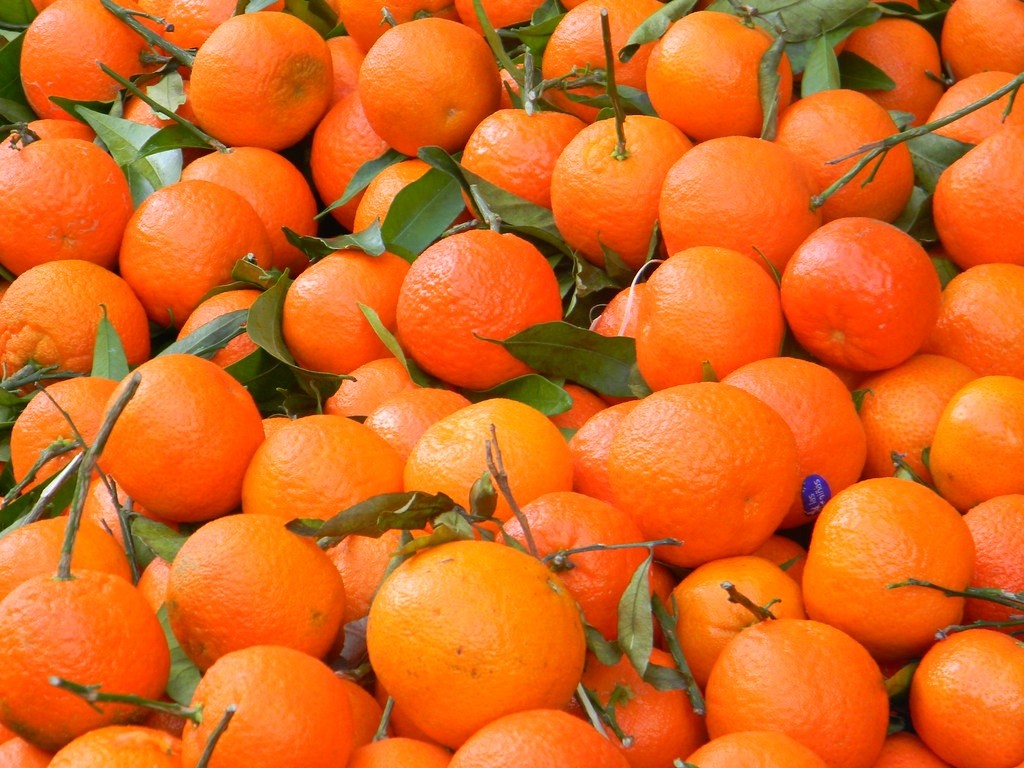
[0,0,1024,768]
